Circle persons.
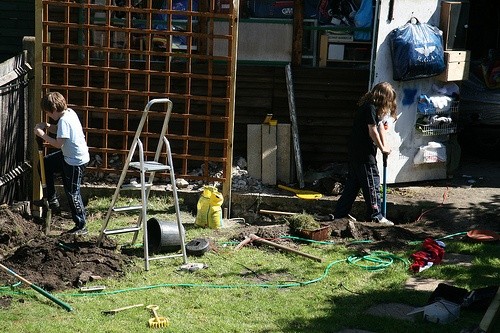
[34,92,90,235]
[334,82,398,225]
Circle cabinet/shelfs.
[328,39,372,64]
[438,50,472,82]
[417,101,459,136]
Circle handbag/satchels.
[388,17,447,82]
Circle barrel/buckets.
[142,216,186,252]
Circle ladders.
[95,99,188,272]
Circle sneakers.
[372,217,395,225]
[335,213,357,222]
[61,226,89,236]
[33,197,60,208]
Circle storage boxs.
[405,282,477,323]
